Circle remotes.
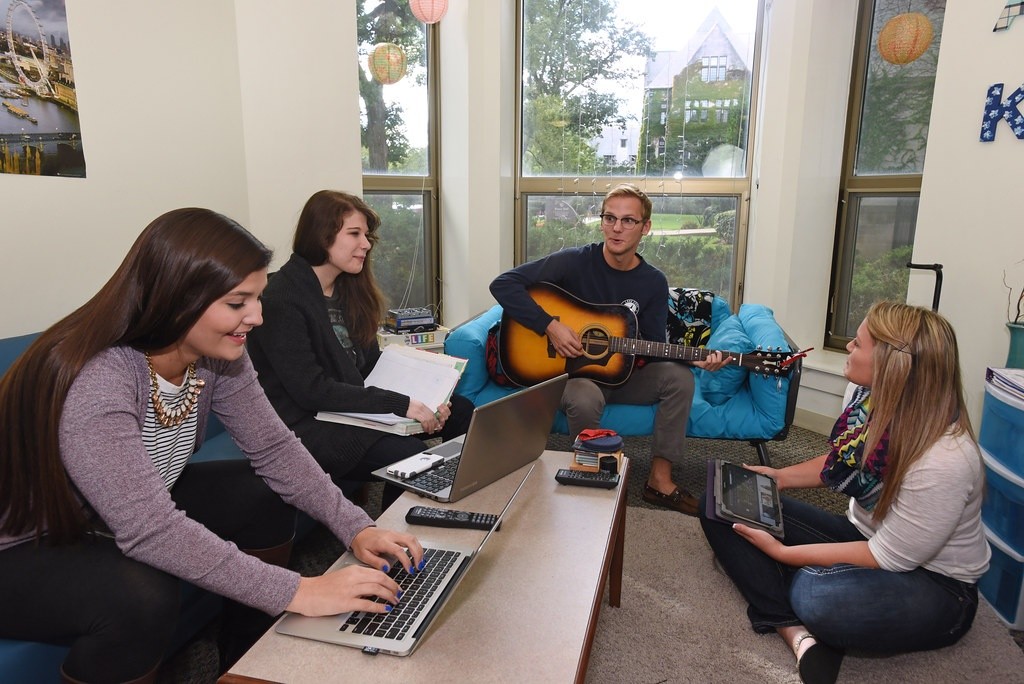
[555,468,620,490]
[405,505,502,533]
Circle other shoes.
[642,480,700,516]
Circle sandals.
[796,633,845,684]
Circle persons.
[0,206,424,684]
[488,183,733,515]
[247,190,452,512]
[698,300,992,684]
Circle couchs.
[0,332,361,684]
[442,288,804,466]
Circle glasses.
[600,214,645,230]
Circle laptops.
[275,462,535,659]
[370,370,570,504]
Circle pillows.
[668,286,714,348]
[698,314,754,407]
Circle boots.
[59,655,167,684]
[218,532,296,674]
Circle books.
[315,343,470,437]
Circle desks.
[215,448,630,683]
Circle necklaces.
[145,352,205,427]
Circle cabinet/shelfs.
[977,372,1024,631]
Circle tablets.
[704,458,785,540]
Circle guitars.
[497,279,807,387]
[485,319,815,388]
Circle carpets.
[422,425,851,519]
